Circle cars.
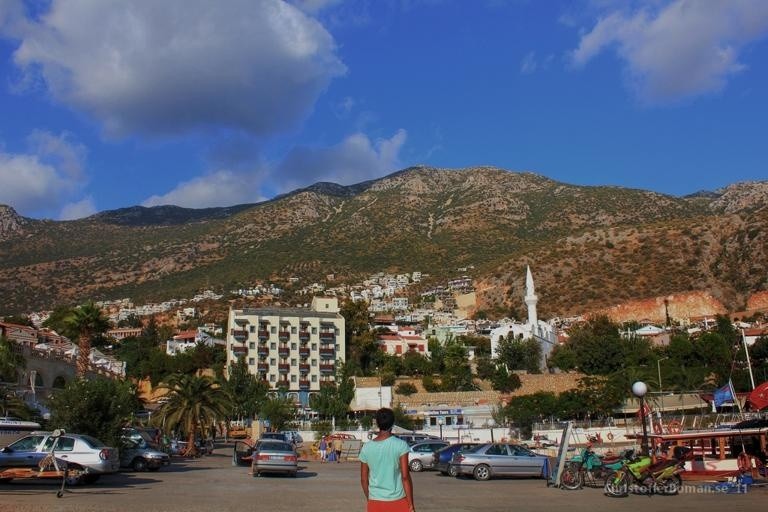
[114,437,170,473]
[432,443,482,479]
[452,444,555,482]
[324,433,356,447]
[230,438,298,479]
[0,431,119,487]
[405,442,449,473]
[259,431,286,442]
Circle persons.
[240,447,257,475]
[357,407,416,512]
[334,436,344,462]
[153,429,161,444]
[211,425,221,442]
[319,435,327,464]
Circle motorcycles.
[603,446,692,496]
[561,442,631,491]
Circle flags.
[748,381,768,410]
[712,384,734,407]
[635,403,650,421]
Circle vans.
[392,432,441,448]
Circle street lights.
[631,381,649,457]
[656,356,669,397]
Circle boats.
[623,328,767,487]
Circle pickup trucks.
[521,435,554,449]
